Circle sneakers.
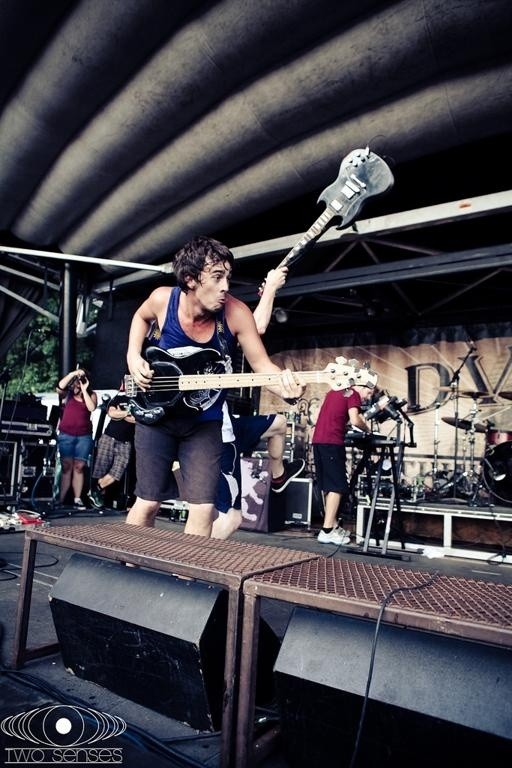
[72,500,88,511]
[317,524,351,546]
[86,486,107,512]
[53,500,65,511]
[269,457,306,495]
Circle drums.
[480,440,512,503]
[484,428,512,452]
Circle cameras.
[78,376,87,383]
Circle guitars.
[123,346,378,426]
[257,146,396,299]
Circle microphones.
[469,340,480,356]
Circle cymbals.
[441,417,486,433]
[456,391,494,399]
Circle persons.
[125,236,304,582]
[87,391,141,510]
[173,267,305,538]
[53,370,98,511]
[312,375,376,544]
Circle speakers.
[272,606,511,768]
[47,554,282,738]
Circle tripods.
[419,420,494,506]
